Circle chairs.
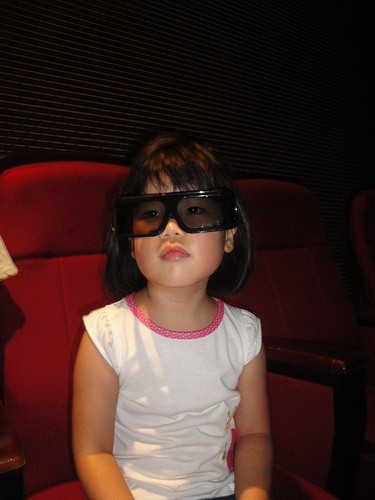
[0,161,375,500]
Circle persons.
[71,131,272,500]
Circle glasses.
[115,189,241,238]
